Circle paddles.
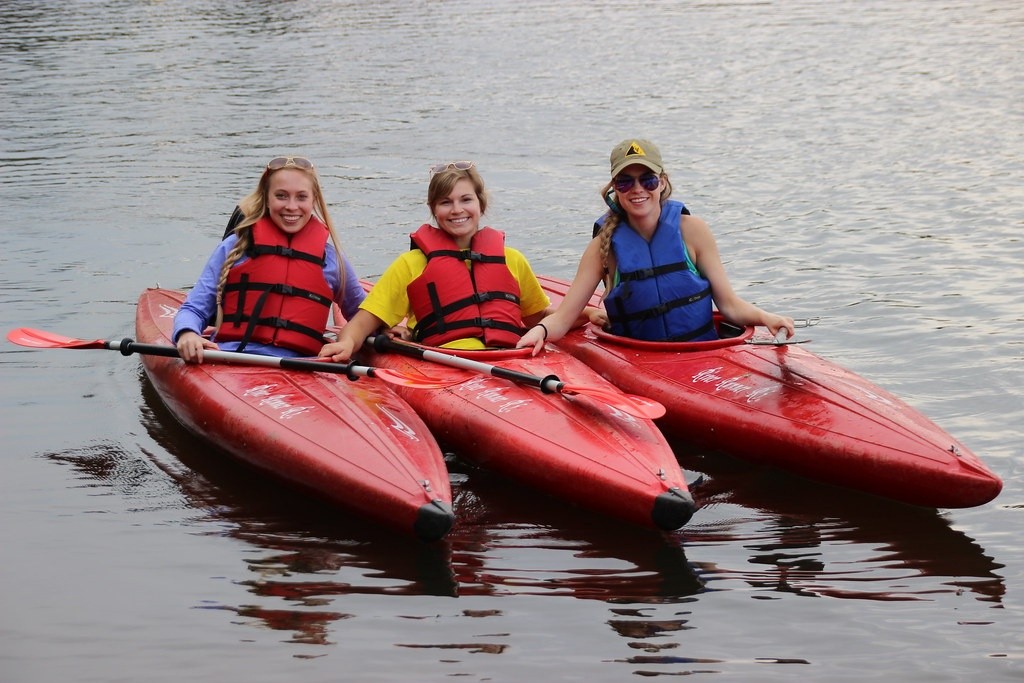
[743,316,822,345]
[324,325,667,420]
[6,326,481,390]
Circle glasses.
[613,173,662,193]
[267,157,313,174]
[429,161,472,183]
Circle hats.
[610,138,664,179]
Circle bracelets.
[535,323,548,341]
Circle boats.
[333,279,694,537]
[532,274,1003,513]
[135,287,457,531]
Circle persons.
[172,158,412,364]
[517,138,795,356]
[319,161,611,362]
